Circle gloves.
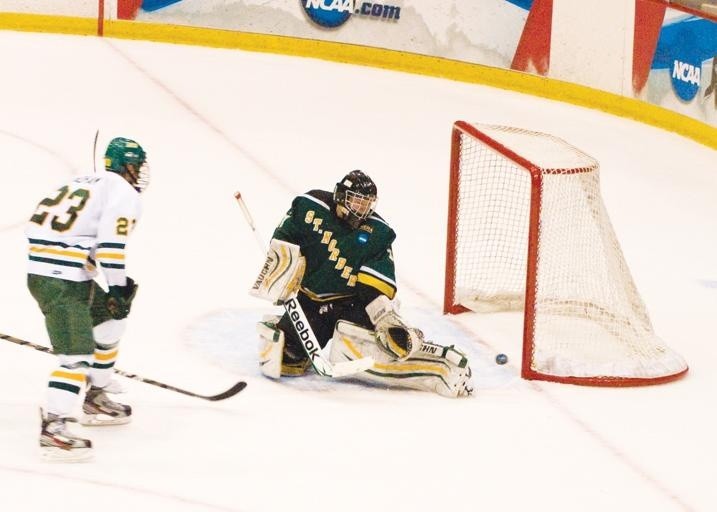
[104,276,139,320]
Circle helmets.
[331,169,378,221]
[103,136,147,174]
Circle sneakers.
[82,384,132,418]
[38,407,91,449]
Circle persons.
[249,169,473,402]
[26,136,147,461]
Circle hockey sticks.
[0,333,247,401]
[235,191,374,379]
